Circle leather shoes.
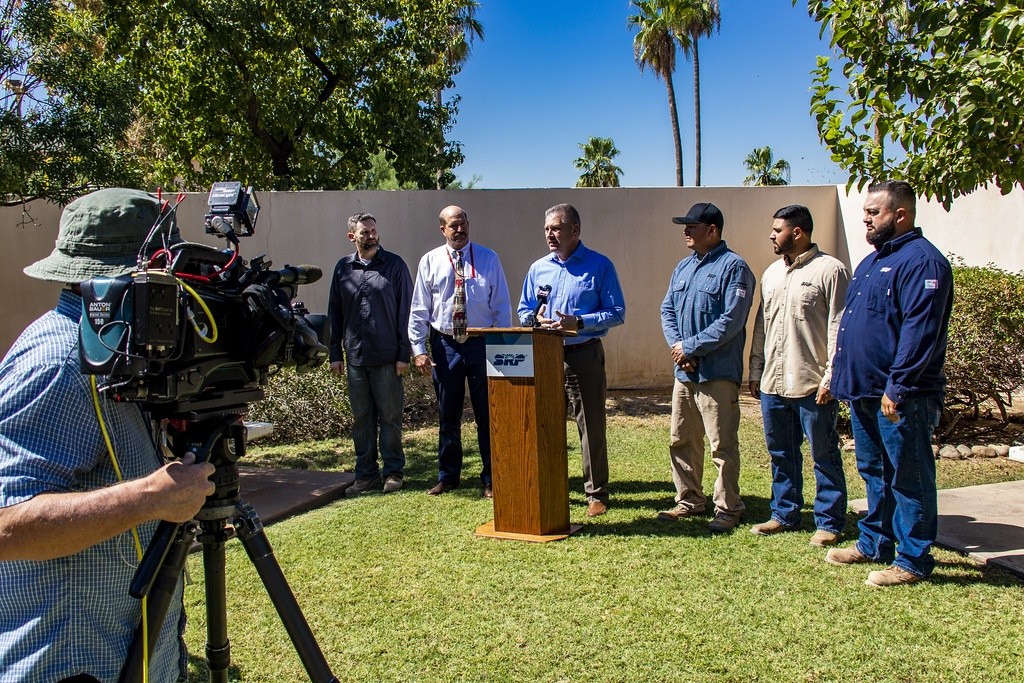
[427,484,448,495]
[482,487,492,498]
[588,501,607,518]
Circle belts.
[565,338,599,349]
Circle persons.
[658,204,756,532]
[330,213,415,497]
[826,182,954,587]
[407,205,513,498]
[0,187,216,683]
[749,205,851,545]
[518,205,625,517]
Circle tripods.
[116,389,340,683]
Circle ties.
[452,251,469,343]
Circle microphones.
[528,284,552,321]
[294,264,322,285]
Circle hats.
[672,203,724,229]
[23,188,217,283]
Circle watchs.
[575,315,584,330]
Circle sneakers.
[658,504,705,521]
[345,473,383,497]
[383,474,402,493]
[809,530,838,547]
[825,543,896,567]
[750,520,786,536]
[865,565,923,587]
[708,513,738,532]
